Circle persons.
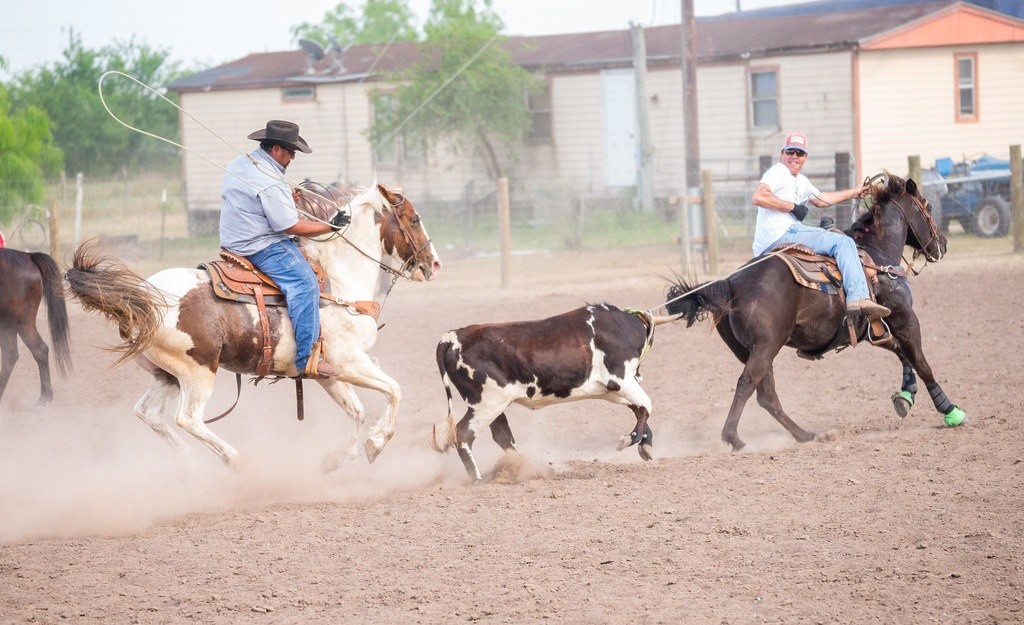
[219,120,351,377]
[752,134,891,317]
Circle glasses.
[280,144,296,157]
[786,150,806,157]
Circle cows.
[428,302,683,482]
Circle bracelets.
[792,204,794,210]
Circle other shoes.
[317,360,339,377]
[846,300,891,316]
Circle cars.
[921,156,1014,241]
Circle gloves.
[791,202,809,222]
[329,211,351,231]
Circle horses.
[0,185,443,474]
[655,169,966,457]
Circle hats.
[246,120,312,153]
[783,134,808,153]
